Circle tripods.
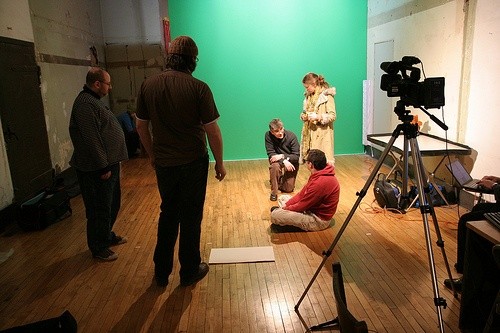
[294,110,458,333]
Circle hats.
[167,36,198,56]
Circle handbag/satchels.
[373,169,404,214]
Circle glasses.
[195,57,199,62]
[302,159,312,165]
[94,79,112,87]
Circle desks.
[367,132,471,210]
[464,221,500,255]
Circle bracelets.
[492,182,498,190]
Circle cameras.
[380,56,446,109]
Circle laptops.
[445,159,484,191]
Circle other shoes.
[110,234,127,244]
[94,248,117,261]
[444,277,462,291]
[154,266,168,287]
[128,150,139,159]
[271,206,279,212]
[181,262,209,286]
[270,194,277,201]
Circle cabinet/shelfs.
[105,43,163,118]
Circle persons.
[133,35,227,284]
[116,110,139,157]
[265,118,301,201]
[67,66,128,262]
[270,149,341,231]
[298,73,336,168]
[443,175,500,290]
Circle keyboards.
[484,212,500,233]
